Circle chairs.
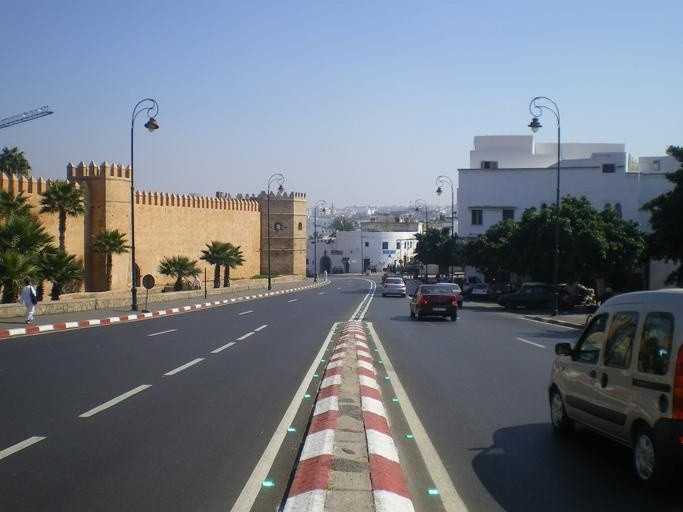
[643,337,659,372]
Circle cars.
[409,276,594,321]
[383,272,406,298]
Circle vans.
[549,288,683,488]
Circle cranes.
[0,105,53,130]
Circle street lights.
[314,200,326,282]
[436,175,454,284]
[268,173,285,290]
[131,98,160,310]
[415,198,428,280]
[528,97,560,316]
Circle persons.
[20,278,37,324]
[192,276,201,290]
[324,269,327,282]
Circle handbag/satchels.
[29,285,37,305]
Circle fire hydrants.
[324,271,327,281]
